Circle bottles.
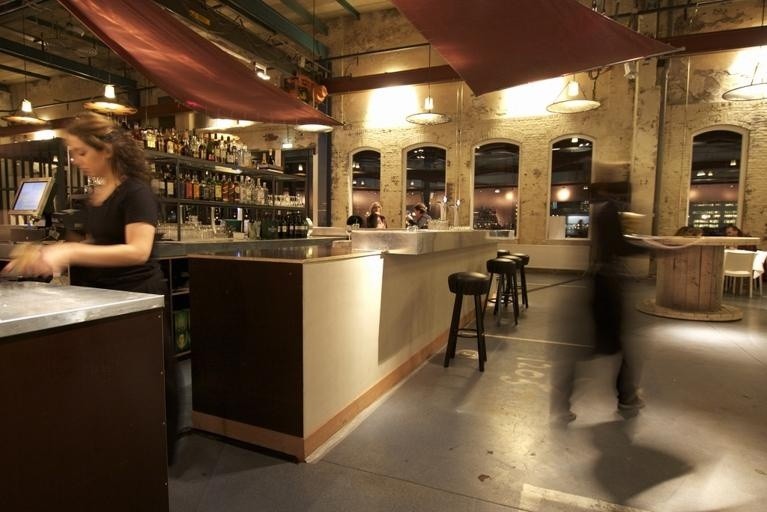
[129,121,254,168]
[268,149,274,165]
[260,152,268,167]
[274,209,308,239]
[249,215,257,239]
[270,188,304,208]
[156,169,269,206]
[244,214,249,232]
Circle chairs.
[721,249,757,298]
[739,250,767,298]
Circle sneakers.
[617,396,646,411]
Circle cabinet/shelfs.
[0,124,310,364]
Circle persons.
[548,196,707,423]
[0,111,182,467]
[366,201,387,229]
[347,215,364,228]
[721,223,756,251]
[407,202,432,229]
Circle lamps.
[545,74,602,115]
[407,44,451,127]
[720,6,767,101]
[83,46,138,116]
[294,1,333,133]
[623,62,638,81]
[201,118,256,129]
[2,0,49,129]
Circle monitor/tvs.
[565,213,589,237]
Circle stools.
[498,255,524,318]
[496,249,509,255]
[443,271,490,371]
[486,259,517,322]
[510,252,529,308]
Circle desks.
[624,234,760,322]
